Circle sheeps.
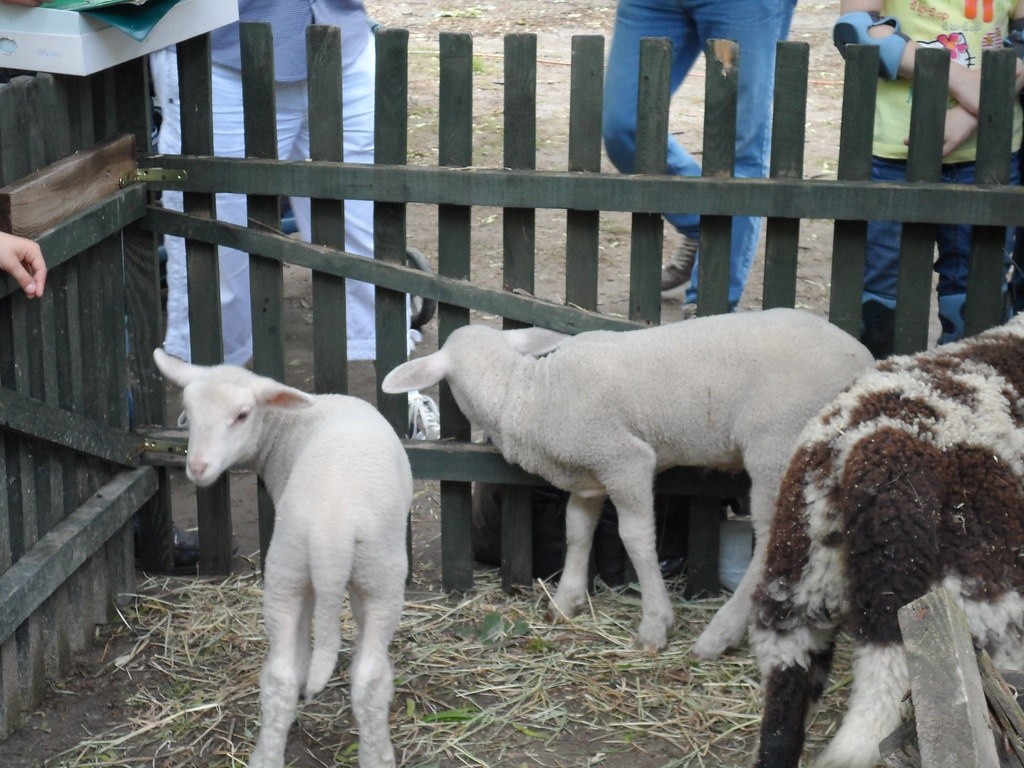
[752,312,1024,768]
[151,348,413,768]
[380,307,874,658]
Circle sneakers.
[681,302,697,321]
[661,236,698,298]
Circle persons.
[0,0,441,477]
[601,0,795,321]
[832,0,1024,361]
[0,233,50,300]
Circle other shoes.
[405,392,440,441]
